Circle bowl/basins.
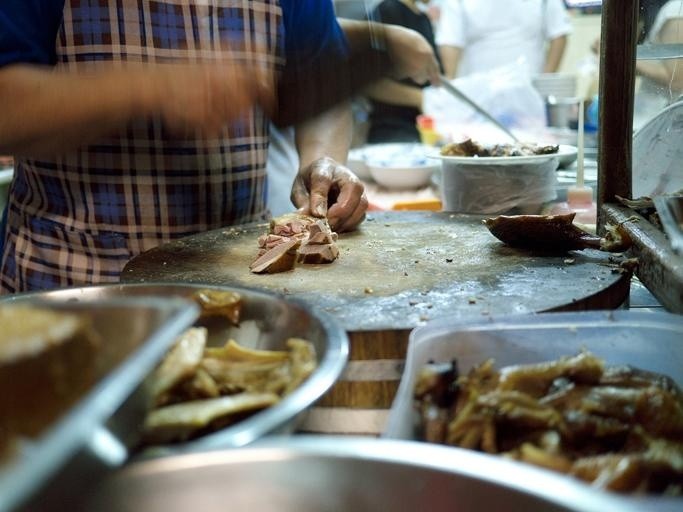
[364,154,439,189]
[1,280,353,456]
[439,148,560,216]
[80,439,660,509]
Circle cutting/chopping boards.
[120,207,631,419]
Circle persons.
[1,0,447,301]
[436,0,573,80]
[591,0,683,132]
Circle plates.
[434,143,581,168]
